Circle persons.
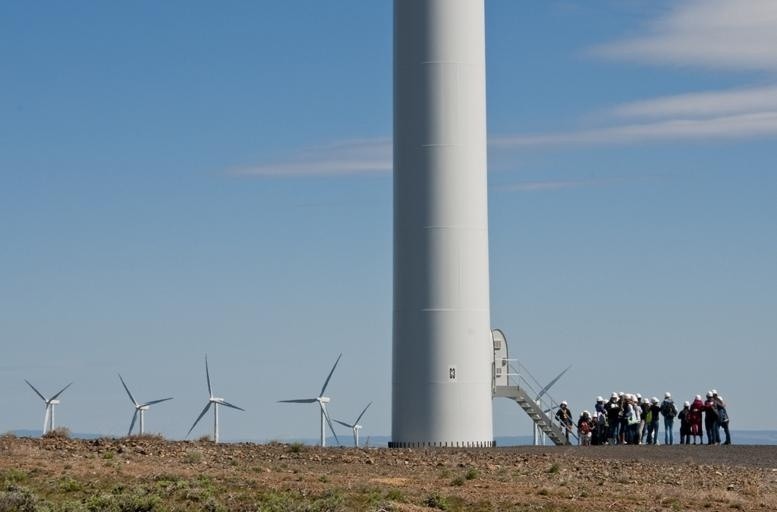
[554,389,732,445]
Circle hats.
[707,391,714,398]
[561,401,567,407]
[596,392,659,404]
[695,395,701,401]
[593,414,597,417]
[684,402,690,407]
[665,392,671,398]
[712,390,718,395]
